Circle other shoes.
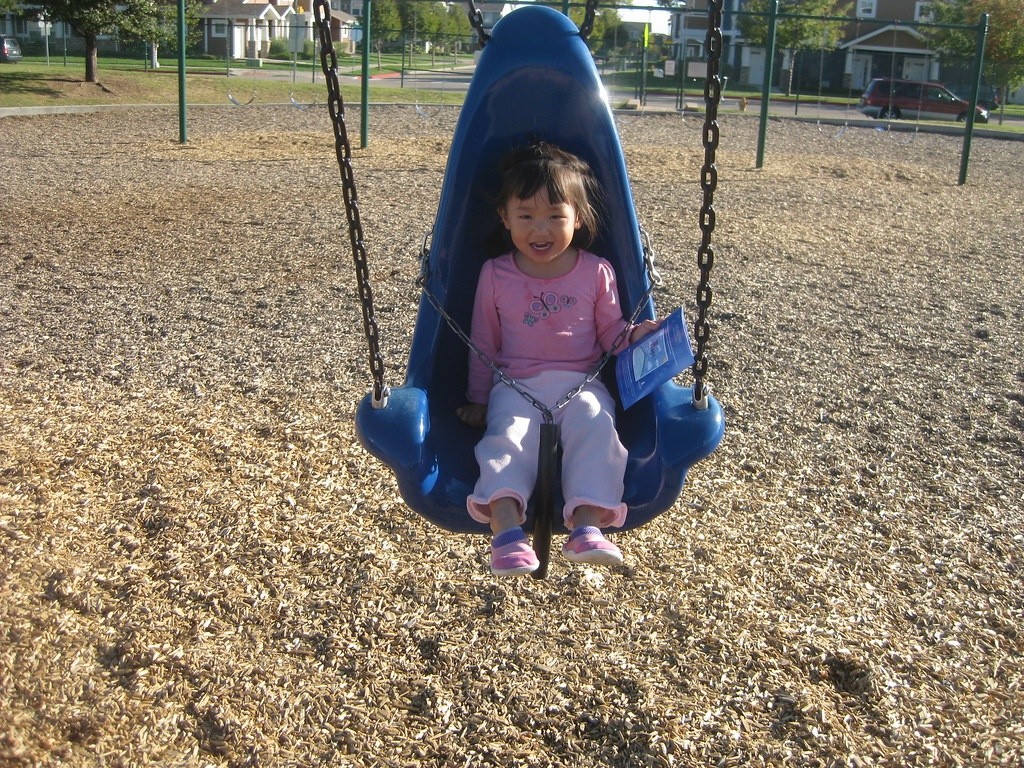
[562,525,623,568]
[490,525,540,576]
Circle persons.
[453,140,669,575]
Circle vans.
[859,78,989,125]
[939,78,1000,111]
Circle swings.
[226,25,257,107]
[816,15,862,138]
[887,19,934,144]
[682,10,716,130]
[290,27,320,111]
[415,1,450,117]
[610,6,649,125]
[309,1,727,580]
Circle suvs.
[0,36,22,64]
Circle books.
[614,306,697,412]
